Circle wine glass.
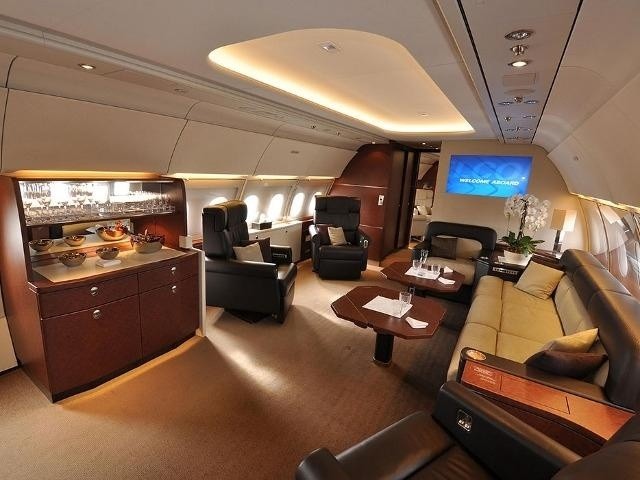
[21,183,175,226]
[421,249,429,271]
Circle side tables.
[487,250,524,282]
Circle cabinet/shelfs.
[0,174,207,404]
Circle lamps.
[549,208,577,252]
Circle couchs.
[446,249,640,448]
[409,215,432,244]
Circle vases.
[503,250,536,267]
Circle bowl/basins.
[28,226,129,252]
[56,239,164,268]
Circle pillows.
[523,350,608,380]
[436,235,483,259]
[328,226,347,246]
[514,261,565,300]
[540,328,599,353]
[240,237,272,262]
[431,235,457,260]
[413,205,428,215]
[532,254,559,264]
[315,224,334,245]
[232,242,264,262]
[523,257,564,272]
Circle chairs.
[308,195,370,280]
[412,221,497,293]
[295,381,640,480]
[201,200,297,324]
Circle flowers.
[499,193,551,257]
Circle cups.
[413,260,440,277]
[399,291,412,319]
